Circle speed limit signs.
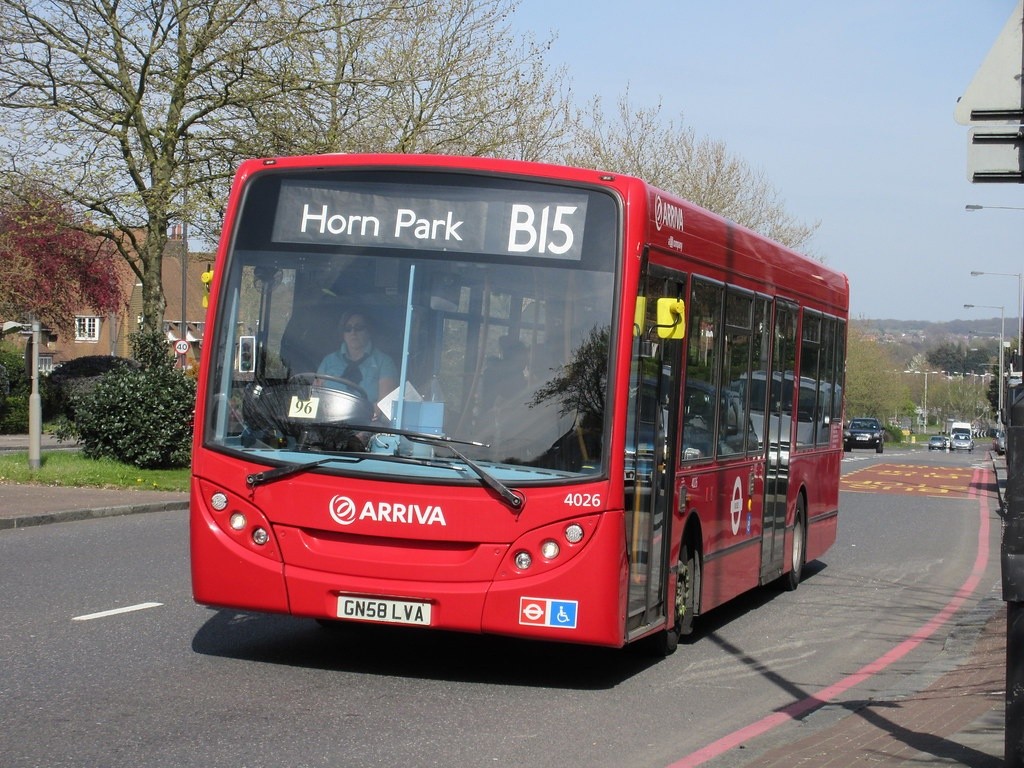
[176,339,190,355]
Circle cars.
[944,437,950,449]
[929,436,945,449]
[900,425,913,435]
[992,429,1007,455]
[950,432,974,452]
[622,355,843,524]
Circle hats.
[499,336,528,361]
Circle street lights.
[970,270,1024,358]
[964,304,1005,426]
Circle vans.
[950,422,973,442]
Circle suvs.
[844,416,886,453]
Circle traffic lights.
[239,335,256,373]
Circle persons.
[312,309,396,445]
[476,331,549,432]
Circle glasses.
[343,324,367,332]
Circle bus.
[188,153,850,657]
